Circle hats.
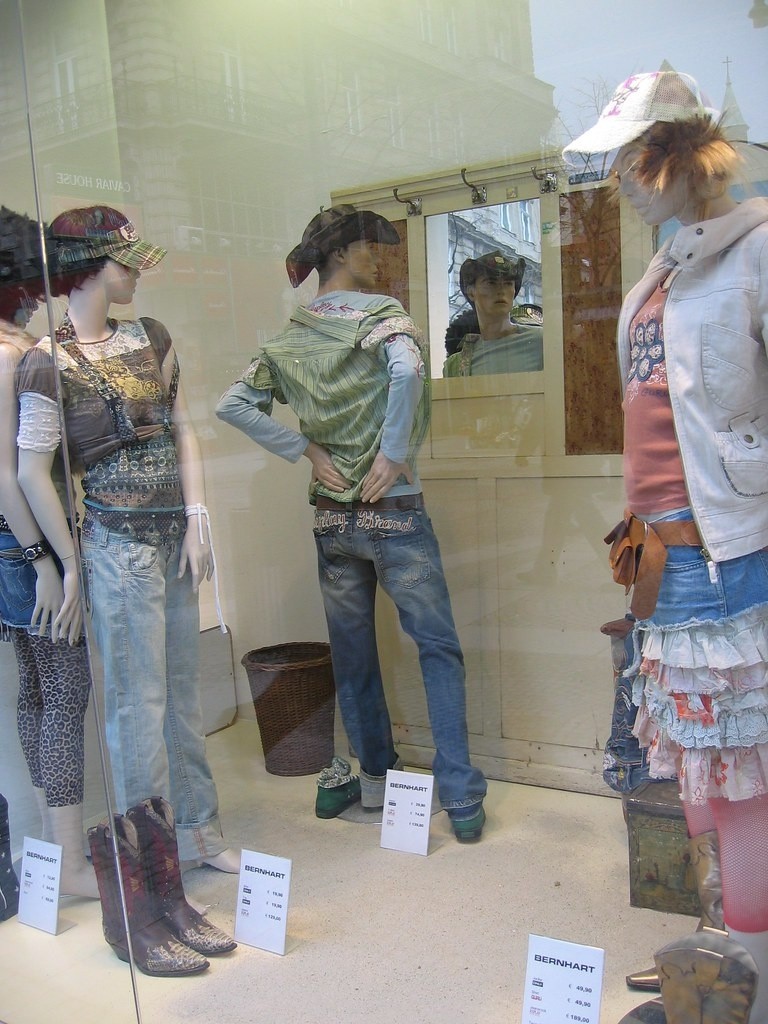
[562,70,704,170]
[459,250,527,288]
[287,203,404,292]
[0,209,52,286]
[46,205,169,277]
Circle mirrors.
[423,195,548,380]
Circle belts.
[308,492,425,514]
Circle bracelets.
[23,540,50,564]
[183,503,227,634]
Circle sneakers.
[445,802,487,845]
[312,756,364,822]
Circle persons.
[0,210,105,898]
[559,66,768,1023]
[214,204,489,850]
[439,250,545,378]
[15,200,244,892]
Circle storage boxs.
[237,640,341,780]
[624,775,722,919]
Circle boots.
[127,792,240,958]
[86,812,210,978]
[0,793,21,922]
